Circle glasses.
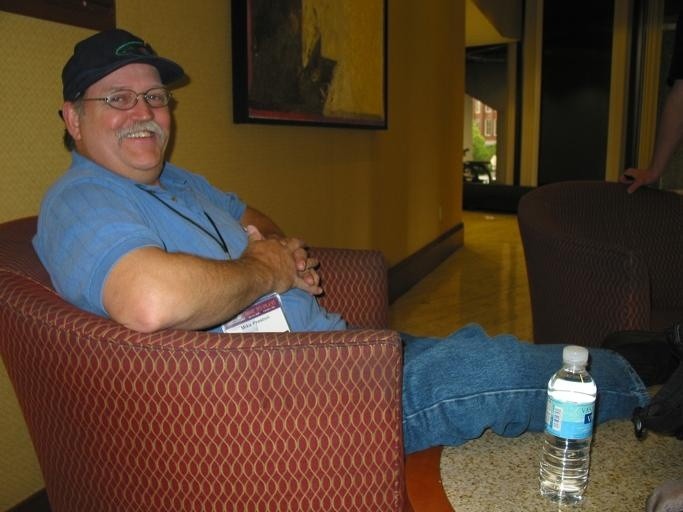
[78,87,173,110]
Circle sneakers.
[615,323,682,386]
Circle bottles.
[537,343,597,506]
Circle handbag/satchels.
[634,360,683,440]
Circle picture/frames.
[231,0,388,131]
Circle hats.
[61,29,189,103]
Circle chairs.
[1,215,406,509]
[515,180,683,346]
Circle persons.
[31,28,682,457]
[619,0,683,194]
